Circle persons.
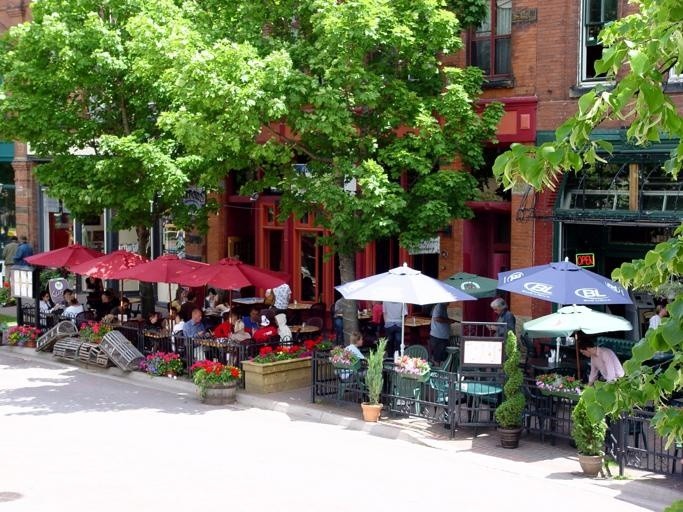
[646,303,669,361]
[1,234,19,286]
[380,301,409,358]
[576,336,626,396]
[370,300,383,338]
[336,330,370,401]
[427,301,462,367]
[485,296,516,388]
[332,294,364,349]
[12,236,33,267]
[36,276,301,370]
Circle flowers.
[8,325,41,346]
[189,359,244,398]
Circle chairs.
[45,296,375,378]
[332,316,683,459]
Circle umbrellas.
[21,241,107,270]
[520,303,633,380]
[110,253,212,284]
[171,255,294,292]
[332,259,480,359]
[495,255,635,307]
[442,270,499,300]
[66,249,153,281]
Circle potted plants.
[569,396,609,476]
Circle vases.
[199,380,235,405]
[25,340,35,346]
